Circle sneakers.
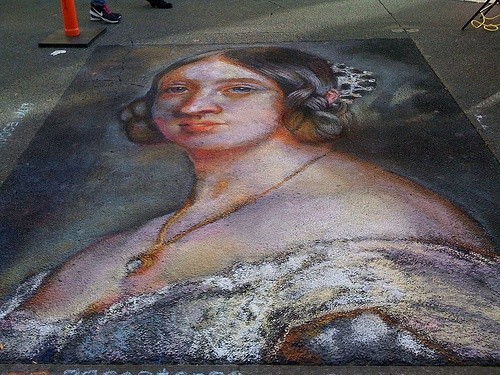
[89,3,122,23]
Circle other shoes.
[149,0,173,9]
